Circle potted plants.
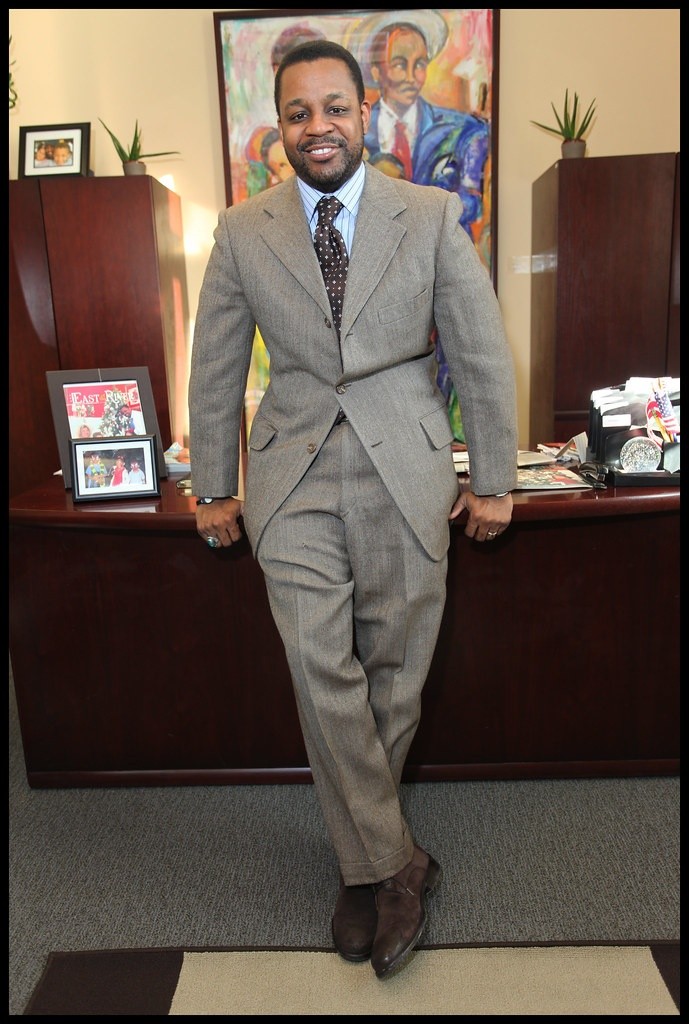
[96,117,181,175]
[529,87,597,159]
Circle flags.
[646,391,680,453]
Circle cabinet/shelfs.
[8,175,192,498]
[528,153,681,448]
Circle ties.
[314,197,349,425]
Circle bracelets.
[495,492,509,497]
[196,497,232,505]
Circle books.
[163,441,192,472]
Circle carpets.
[21,940,680,1015]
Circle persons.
[110,453,145,486]
[86,452,107,488]
[119,404,146,436]
[190,41,517,980]
[34,139,72,167]
[77,423,104,439]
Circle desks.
[9,449,680,790]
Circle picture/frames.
[68,434,162,506]
[45,366,168,491]
[213,8,518,450]
[18,122,91,179]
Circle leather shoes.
[370,844,442,981]
[332,870,381,962]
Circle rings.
[207,536,218,548]
[487,532,497,537]
